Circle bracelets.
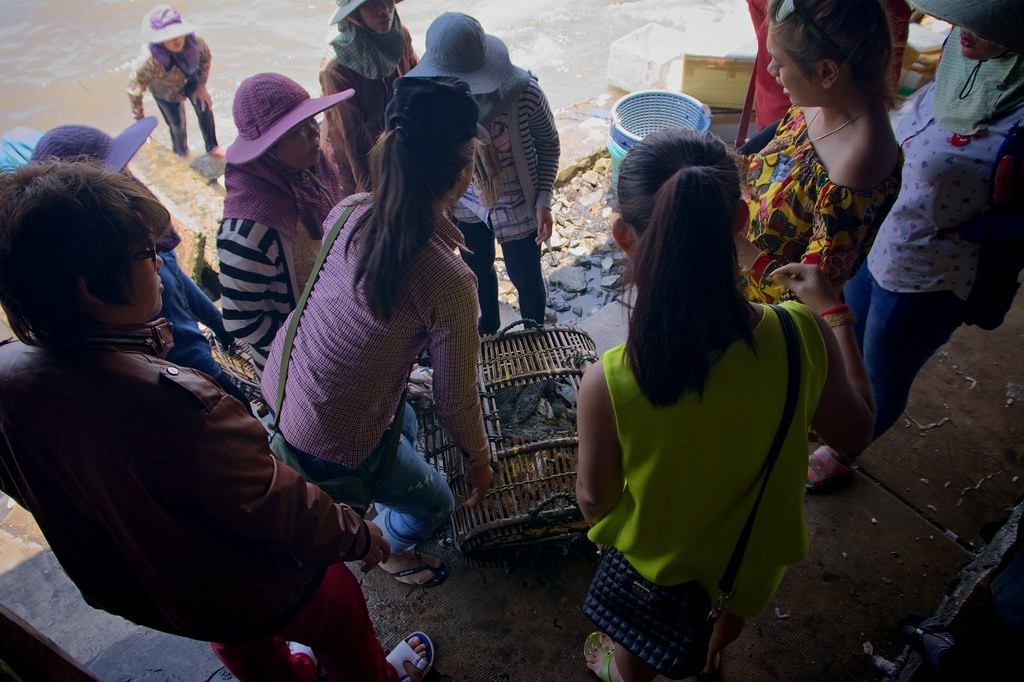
[821,305,847,316]
[823,313,856,327]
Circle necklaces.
[791,111,864,155]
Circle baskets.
[610,91,712,145]
[606,142,630,196]
[413,318,598,561]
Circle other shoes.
[208,146,225,158]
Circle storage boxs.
[607,14,756,142]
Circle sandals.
[386,631,434,682]
[393,548,448,588]
[804,447,857,495]
[584,632,616,682]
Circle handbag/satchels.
[266,427,373,520]
[581,546,713,680]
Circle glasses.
[776,0,849,67]
[132,237,157,261]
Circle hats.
[225,72,356,164]
[909,1,1024,134]
[331,0,406,81]
[148,9,193,45]
[32,116,158,173]
[385,76,492,159]
[405,12,508,96]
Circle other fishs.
[488,377,578,504]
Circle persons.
[736,0,1024,490]
[217,73,356,387]
[260,75,496,587]
[401,12,560,335]
[127,4,225,158]
[29,115,253,414]
[316,0,418,196]
[0,157,434,682]
[574,127,878,682]
